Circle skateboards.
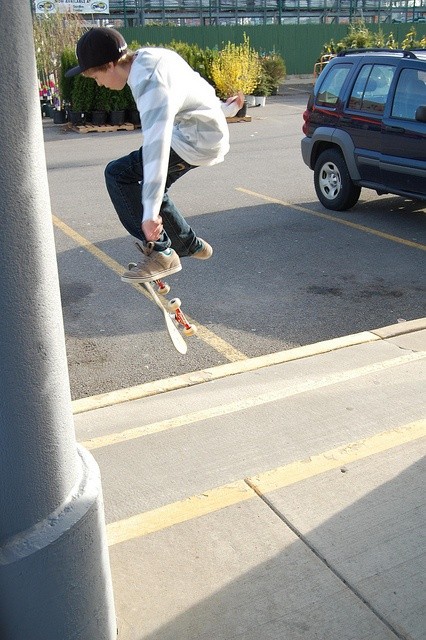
[128,259,197,353]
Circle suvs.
[300,48,426,212]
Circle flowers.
[52,83,63,111]
[39,81,47,96]
[45,78,55,106]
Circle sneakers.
[121,241,183,284]
[189,237,213,260]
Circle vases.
[43,105,52,117]
[43,95,47,99]
[54,111,66,124]
[48,106,57,118]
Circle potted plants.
[235,30,260,107]
[210,32,261,117]
[255,68,271,107]
[69,75,94,125]
[59,45,79,120]
[110,89,127,125]
[264,50,285,96]
[127,86,141,125]
[90,84,110,125]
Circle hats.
[64,26,128,78]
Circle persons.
[65,28,232,284]
